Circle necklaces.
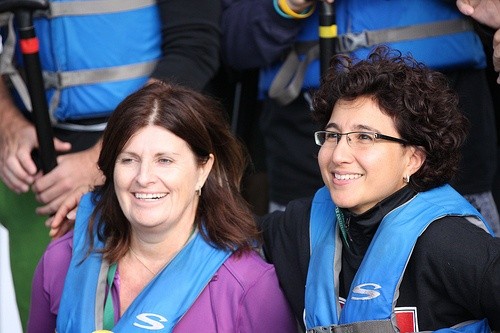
[128,244,157,277]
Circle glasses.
[314,130,410,149]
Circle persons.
[0,0,500,332]
